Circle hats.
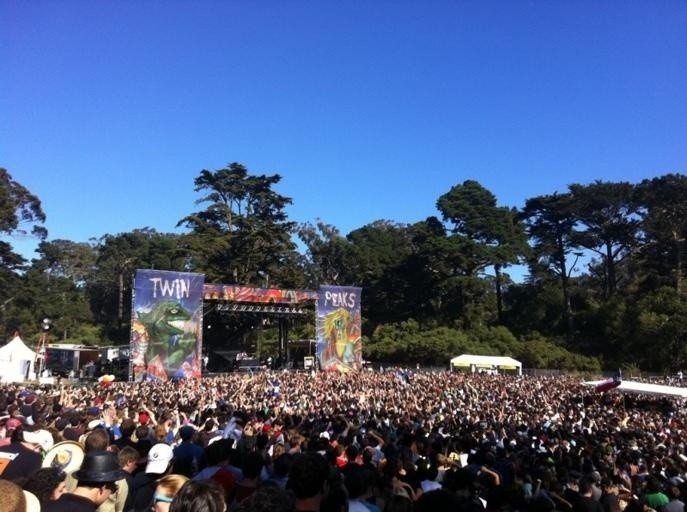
[6,393,175,484]
